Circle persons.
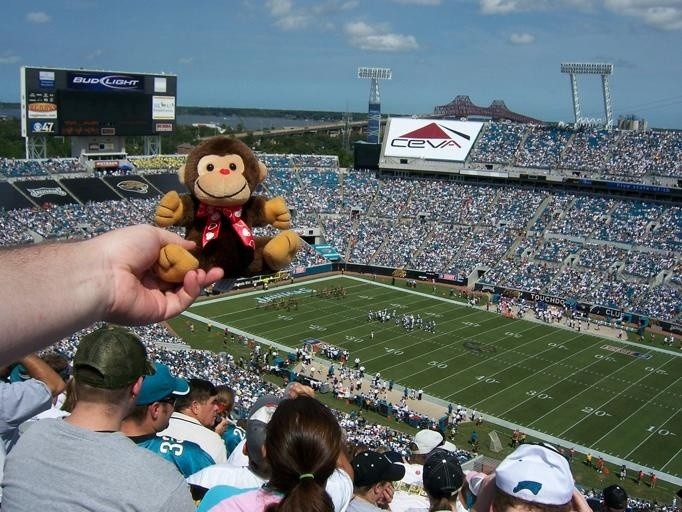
[1,223,224,372]
[254,119,681,288]
[0,285,682,511]
[0,153,188,248]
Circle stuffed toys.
[148,136,300,286]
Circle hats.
[73,324,157,388]
[136,363,191,406]
[351,429,575,505]
[246,398,283,465]
[603,484,627,502]
